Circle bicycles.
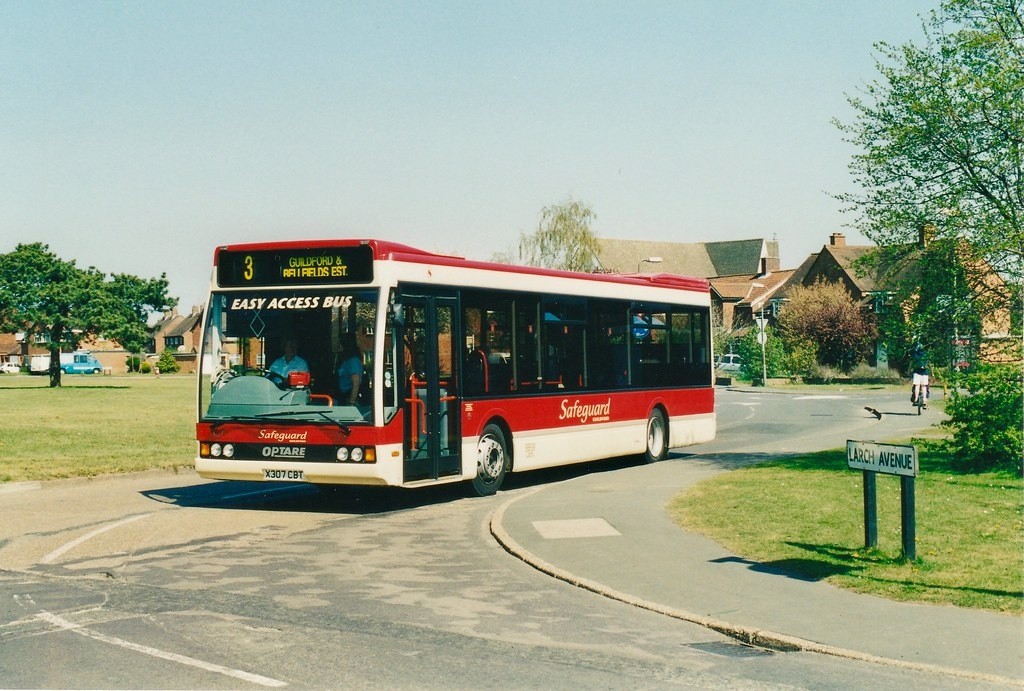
[913,383,927,416]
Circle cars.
[714,353,754,373]
[0,362,22,373]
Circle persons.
[911,343,930,410]
[337,333,364,406]
[265,335,309,378]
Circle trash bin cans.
[154,367,159,375]
[104,369,111,375]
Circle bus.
[195,239,715,496]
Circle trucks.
[30,351,103,375]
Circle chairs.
[486,353,511,395]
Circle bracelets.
[349,402,355,404]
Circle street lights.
[638,256,664,273]
